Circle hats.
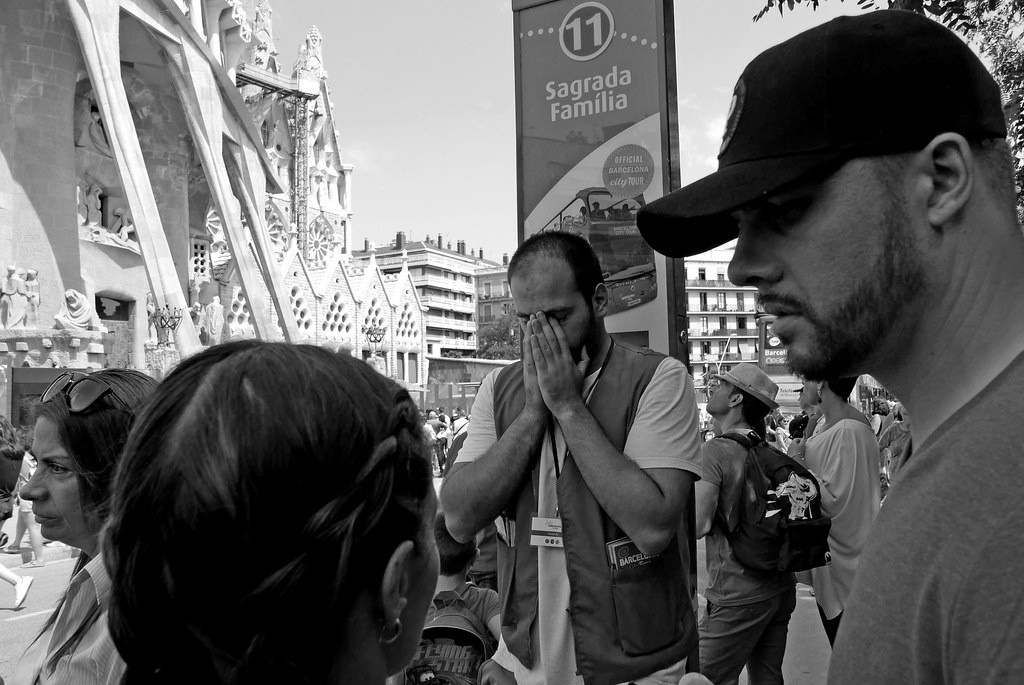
[637,11,1006,258]
[713,362,780,410]
[793,386,804,392]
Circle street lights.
[702,332,738,385]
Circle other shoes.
[3,545,20,553]
[46,541,66,547]
[0,533,8,547]
[20,561,44,568]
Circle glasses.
[39,371,135,420]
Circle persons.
[103,340,438,685]
[112,207,134,241]
[190,296,224,346]
[73,97,112,158]
[0,369,160,685]
[54,289,108,334]
[0,265,40,329]
[394,512,502,685]
[635,9,1024,685]
[414,404,473,477]
[77,174,103,226]
[439,230,703,685]
[146,292,157,343]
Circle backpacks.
[713,430,833,572]
[402,582,496,685]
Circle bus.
[523,186,658,316]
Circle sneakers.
[14,576,33,607]
